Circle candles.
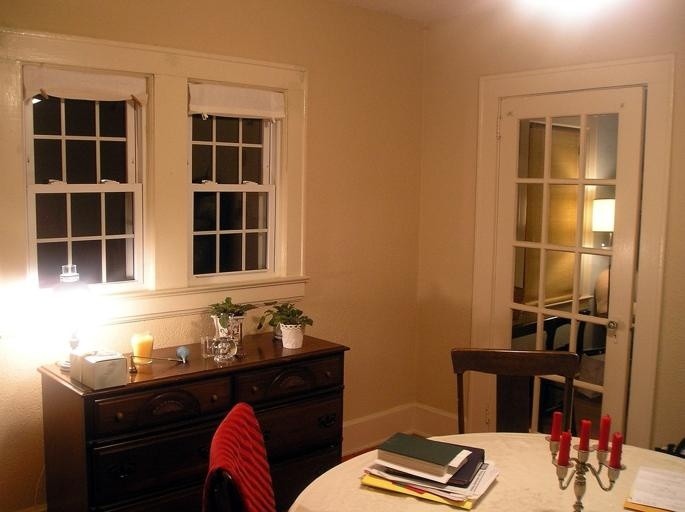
[549,410,624,467]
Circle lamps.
[587,198,616,248]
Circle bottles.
[211,337,240,362]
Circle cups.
[131,334,155,365]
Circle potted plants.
[210,297,257,342]
[258,301,295,339]
[276,308,313,350]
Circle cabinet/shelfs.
[38,330,352,512]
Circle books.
[362,432,501,511]
[622,465,685,512]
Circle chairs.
[450,347,581,434]
[203,400,279,512]
